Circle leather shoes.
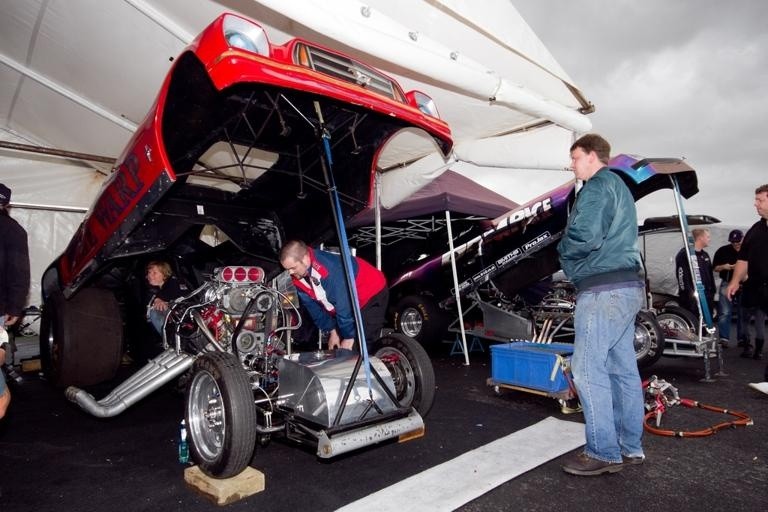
[558,443,645,476]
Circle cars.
[39,11,455,480]
[39,11,723,480]
[336,154,726,388]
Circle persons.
[280,239,390,356]
[556,133,646,477]
[1,184,30,419]
[145,260,198,343]
[725,184,768,400]
[674,227,714,327]
[710,230,750,348]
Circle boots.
[718,340,765,360]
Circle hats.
[728,229,743,242]
